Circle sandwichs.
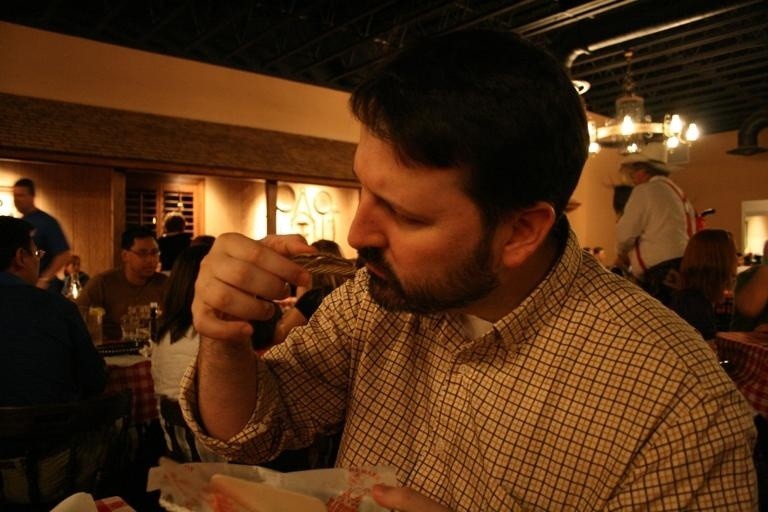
[209,474,328,512]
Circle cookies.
[294,253,357,280]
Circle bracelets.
[37,276,51,284]
[760,258,768,264]
[759,265,768,273]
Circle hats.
[620,142,667,167]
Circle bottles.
[119,302,163,342]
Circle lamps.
[561,48,704,158]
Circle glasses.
[25,250,46,257]
[127,249,161,256]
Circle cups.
[87,307,103,345]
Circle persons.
[157,211,192,272]
[736,253,745,265]
[77,227,171,348]
[11,177,72,291]
[604,140,700,308]
[730,239,768,332]
[172,2,761,511]
[663,227,740,355]
[0,215,115,511]
[274,241,345,344]
[59,254,90,295]
[151,236,216,458]
[593,246,610,271]
[583,247,594,254]
